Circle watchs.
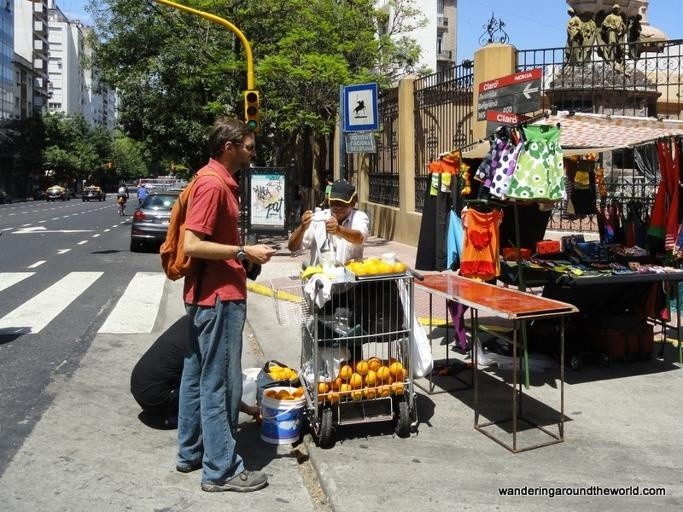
[236,246,246,261]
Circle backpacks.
[160,172,225,280]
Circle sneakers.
[176,461,268,492]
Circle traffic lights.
[244,90,260,136]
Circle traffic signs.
[476,66,541,121]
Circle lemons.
[348,259,406,274]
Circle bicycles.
[116,196,127,215]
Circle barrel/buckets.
[260,386,307,445]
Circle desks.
[414,273,579,453]
[505,250,682,378]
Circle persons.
[602,4,623,54]
[137,184,149,204]
[288,179,369,361]
[293,185,304,223]
[319,174,334,209]
[130,315,260,429]
[117,183,129,207]
[176,117,277,492]
[567,8,582,60]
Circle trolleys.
[269,253,422,449]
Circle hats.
[329,179,357,204]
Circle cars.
[46,185,70,202]
[81,184,105,202]
[129,190,182,253]
